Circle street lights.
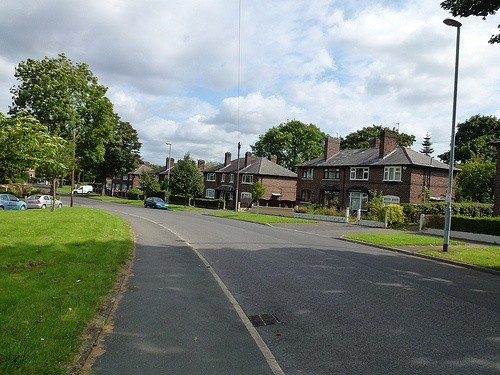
[442,18,463,252]
[165,142,172,203]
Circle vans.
[72,185,93,195]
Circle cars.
[144,197,169,210]
[0,194,62,210]
